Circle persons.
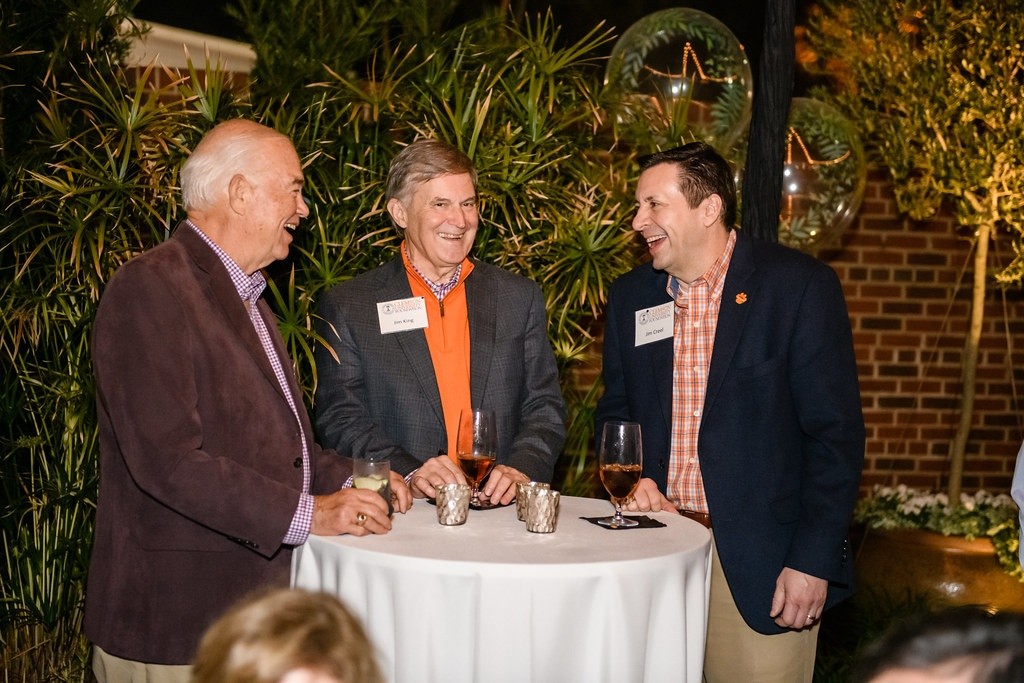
[829,605,1024,683]
[311,138,570,509]
[88,118,413,683]
[189,584,384,683]
[596,139,866,683]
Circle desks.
[291,490,714,683]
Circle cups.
[352,458,391,504]
[435,483,471,526]
[524,489,560,533]
[515,481,550,522]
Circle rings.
[624,496,635,507]
[357,510,366,526]
[806,615,817,620]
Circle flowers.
[855,483,1024,584]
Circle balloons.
[723,97,868,257]
[603,6,755,163]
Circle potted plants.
[799,0,1024,615]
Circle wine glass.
[455,409,497,508]
[597,422,643,528]
